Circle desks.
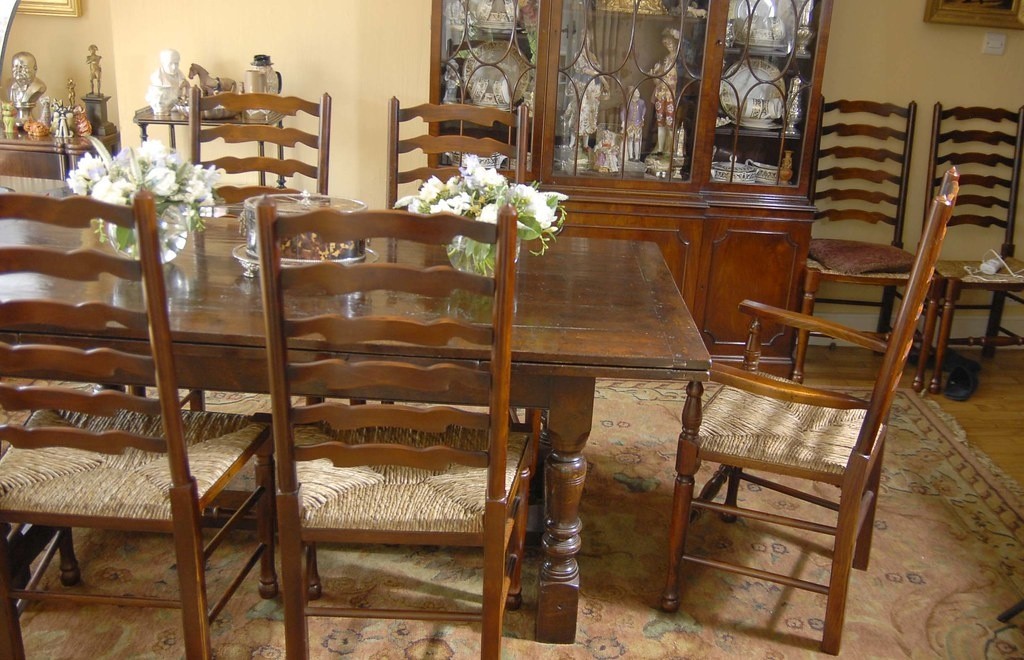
[132,105,287,189]
[0,123,120,182]
[0,174,713,643]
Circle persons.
[8,51,46,126]
[443,24,684,174]
[145,49,187,116]
[39,45,102,139]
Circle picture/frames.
[922,0,1024,30]
[14,0,81,17]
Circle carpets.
[1,377,1024,660]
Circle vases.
[107,204,187,265]
[445,234,520,279]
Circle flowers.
[393,151,570,277]
[65,133,227,261]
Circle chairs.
[791,93,948,393]
[351,95,541,433]
[921,101,1024,394]
[256,194,535,660]
[0,188,279,660]
[680,165,959,656]
[187,85,331,413]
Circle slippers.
[944,365,978,401]
[927,346,982,372]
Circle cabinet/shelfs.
[427,0,833,379]
